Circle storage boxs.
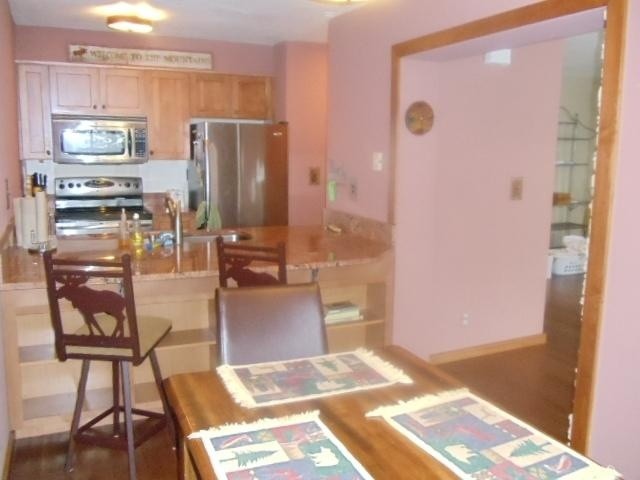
[547,247,588,280]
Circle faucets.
[171,245,185,273]
[167,199,184,246]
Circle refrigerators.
[187,121,288,226]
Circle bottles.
[130,212,145,261]
[119,208,131,250]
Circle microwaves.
[51,114,149,165]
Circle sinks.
[183,230,254,245]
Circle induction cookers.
[52,207,152,220]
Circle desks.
[159,345,624,480]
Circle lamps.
[105,15,153,33]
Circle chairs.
[43,249,177,480]
[215,235,287,288]
[215,284,329,366]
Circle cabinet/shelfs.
[18,63,55,162]
[49,64,148,119]
[146,71,191,161]
[0,257,394,441]
[191,73,272,119]
[550,105,591,248]
